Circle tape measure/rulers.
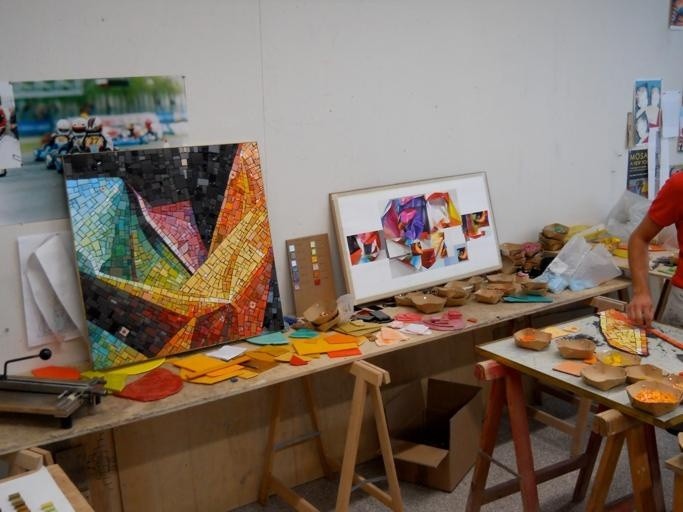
[185,356,251,379]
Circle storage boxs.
[374,377,484,495]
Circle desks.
[465,310,682,512]
[0,265,635,489]
[543,223,683,306]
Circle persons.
[635,86,648,117]
[51,118,69,137]
[85,116,113,151]
[635,116,648,144]
[128,123,135,137]
[627,169,683,330]
[144,117,153,132]
[644,88,661,127]
[69,119,86,142]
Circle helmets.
[56,119,71,135]
[71,116,87,137]
[84,115,104,136]
[144,118,152,128]
[127,122,134,130]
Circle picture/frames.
[329,171,503,307]
